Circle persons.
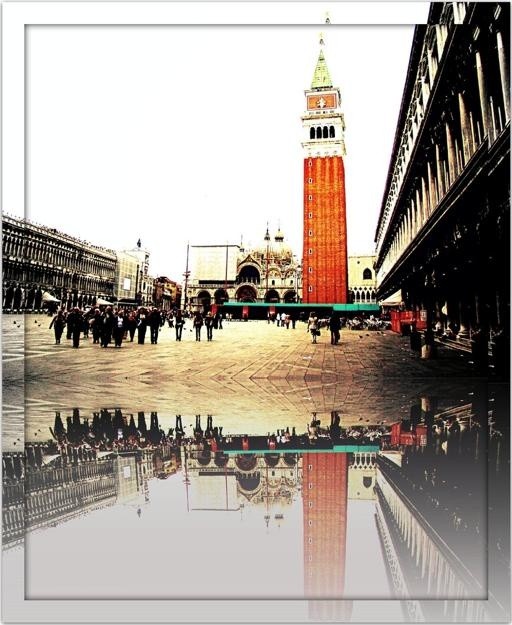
[49,305,232,348]
[49,407,232,452]
[267,411,381,446]
[267,310,374,344]
